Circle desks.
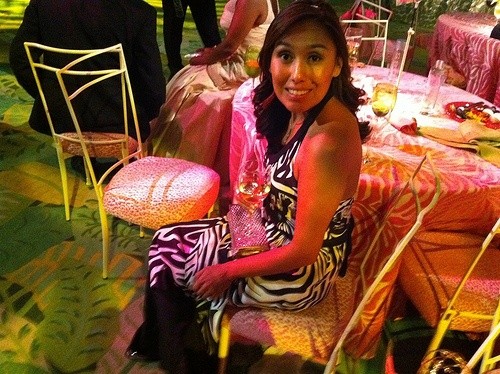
[427,12,500,109]
[229,64,500,359]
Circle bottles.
[422,60,446,112]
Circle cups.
[386,39,406,82]
[343,29,363,70]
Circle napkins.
[417,117,500,167]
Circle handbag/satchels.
[226,202,269,256]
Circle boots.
[124,284,208,374]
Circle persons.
[142,0,280,199]
[162,0,222,83]
[125,0,371,374]
[10,0,165,178]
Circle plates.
[445,102,500,130]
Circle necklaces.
[296,121,304,125]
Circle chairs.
[23,0,500,374]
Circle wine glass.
[366,83,398,147]
[244,32,267,90]
